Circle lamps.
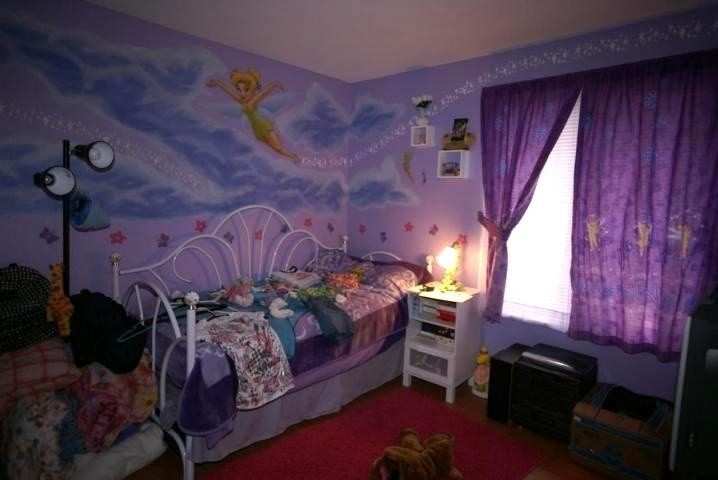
[32,138,117,297]
[436,234,465,293]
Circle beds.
[109,202,436,480]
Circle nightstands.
[402,280,481,404]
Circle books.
[416,297,456,348]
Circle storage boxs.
[567,380,675,480]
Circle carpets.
[191,383,556,480]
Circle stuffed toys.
[45,263,75,342]
[368,426,461,478]
[467,344,491,386]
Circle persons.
[204,66,305,165]
